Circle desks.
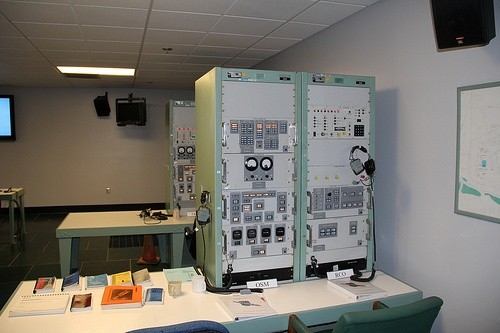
[0,268,424,333]
[55,208,196,277]
[0,187,27,248]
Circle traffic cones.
[136,235,161,265]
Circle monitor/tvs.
[0,95,16,141]
[116,98,147,125]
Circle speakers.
[94,96,110,116]
[431,0,496,49]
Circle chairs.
[124,320,231,333]
[286,294,445,333]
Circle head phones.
[195,190,211,225]
[348,145,376,175]
